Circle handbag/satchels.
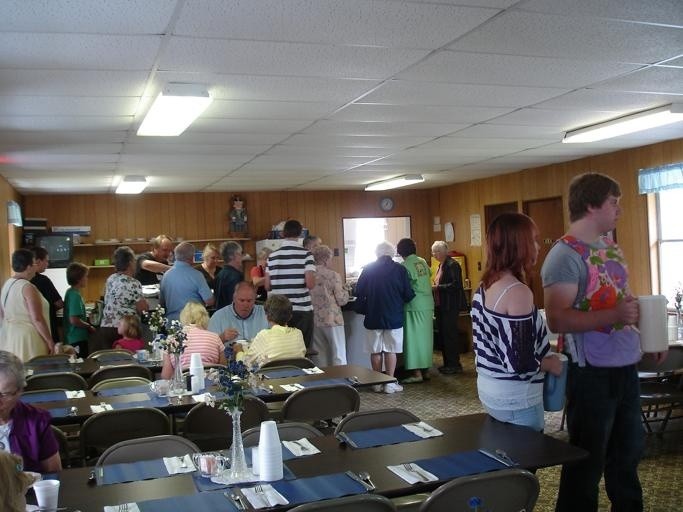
[90,300,104,327]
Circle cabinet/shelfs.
[71,236,255,269]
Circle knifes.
[339,432,357,448]
[345,470,373,490]
[222,491,239,511]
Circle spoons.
[496,449,514,464]
[70,406,78,415]
[233,494,245,511]
[335,434,346,445]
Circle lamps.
[562,103,682,143]
[363,175,423,191]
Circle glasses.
[0,387,20,401]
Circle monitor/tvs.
[35,233,72,267]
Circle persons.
[468,211,568,435]
[537,169,671,510]
[0,451,31,512]
[355,238,464,393]
[2,247,150,364]
[252,220,348,366]
[0,351,66,476]
[137,234,306,379]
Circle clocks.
[379,197,394,213]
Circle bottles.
[189,376,200,393]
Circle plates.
[147,357,161,361]
[210,467,259,484]
[25,504,38,511]
[21,471,40,486]
[167,390,193,398]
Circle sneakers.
[385,381,403,394]
[371,384,383,392]
[401,376,422,383]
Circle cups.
[189,354,204,389]
[629,295,668,352]
[257,421,283,480]
[543,352,568,411]
[135,350,148,362]
[250,447,260,475]
[193,451,224,481]
[668,316,678,341]
[32,479,60,508]
[150,379,185,396]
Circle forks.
[118,504,128,511]
[254,484,271,506]
[412,423,432,432]
[99,401,106,411]
[357,471,375,488]
[403,463,429,481]
